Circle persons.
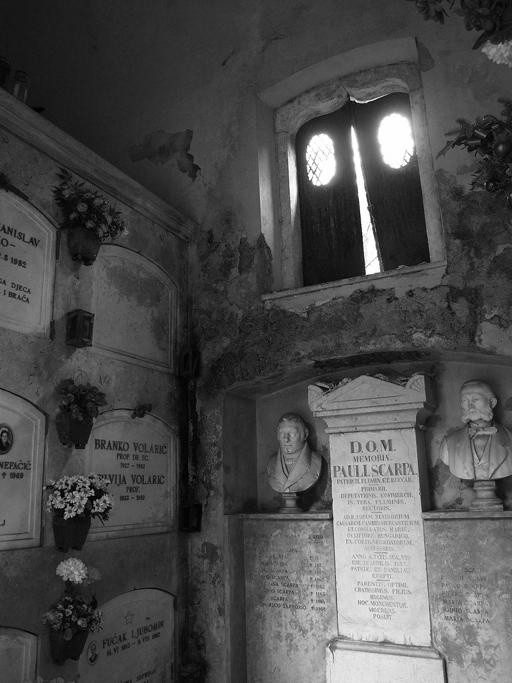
[440,379,512,480]
[265,412,323,493]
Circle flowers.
[40,557,105,641]
[48,167,127,242]
[42,471,113,529]
[57,377,107,423]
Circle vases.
[66,227,102,266]
[56,414,94,451]
[52,515,91,552]
[47,629,90,667]
